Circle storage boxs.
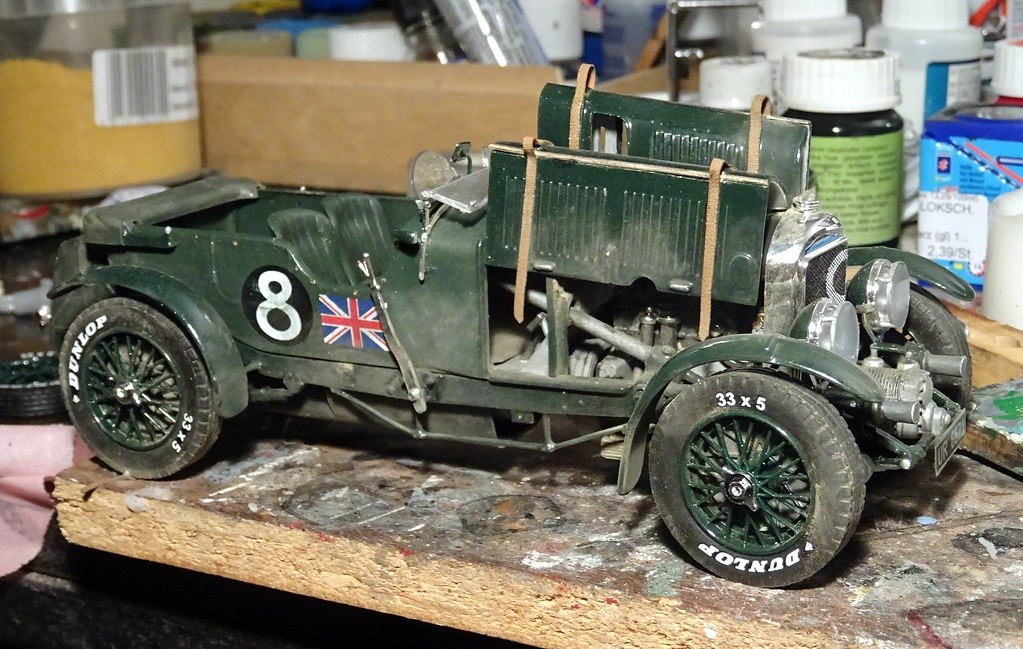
[197,51,713,194]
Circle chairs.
[266,192,415,285]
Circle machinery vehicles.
[52,59,975,589]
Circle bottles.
[1,0,1023,248]
